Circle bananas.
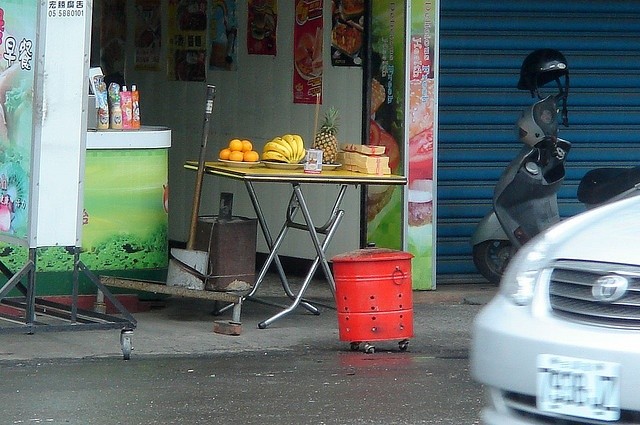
[261,133,305,163]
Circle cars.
[470,183,640,425]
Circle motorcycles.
[470,69,640,286]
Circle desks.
[182,159,407,329]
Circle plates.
[261,161,304,169]
[218,159,260,168]
[322,162,342,171]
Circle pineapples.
[311,108,341,163]
